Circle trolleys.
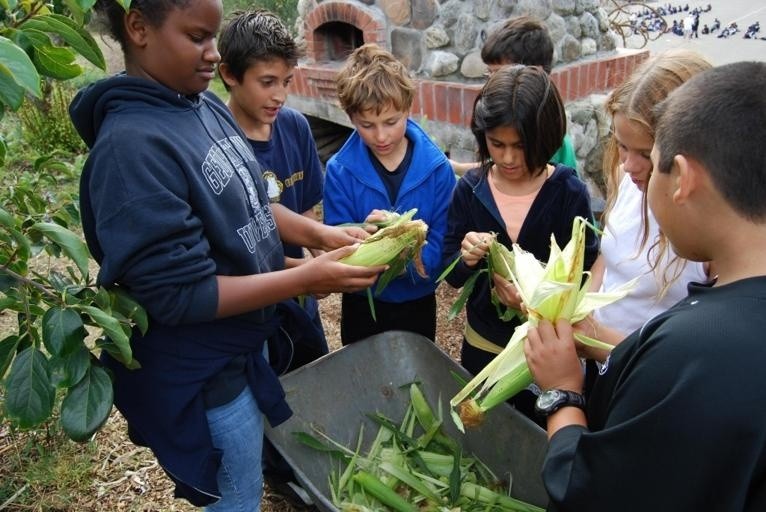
[260,328,550,512]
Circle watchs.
[535,387,587,416]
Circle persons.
[68,0,392,512]
[630,3,760,39]
[522,61,766,512]
[572,49,717,371]
[217,9,327,512]
[448,15,583,177]
[439,63,597,431]
[322,43,458,346]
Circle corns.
[511,243,555,308]
[336,216,426,272]
[490,239,521,284]
